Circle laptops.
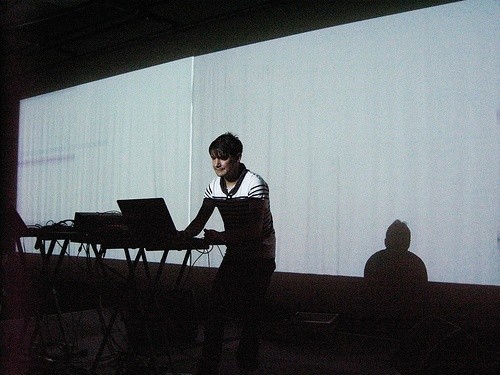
[117,198,177,230]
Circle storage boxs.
[293,311,339,343]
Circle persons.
[176,131,276,375]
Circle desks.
[13,228,208,375]
